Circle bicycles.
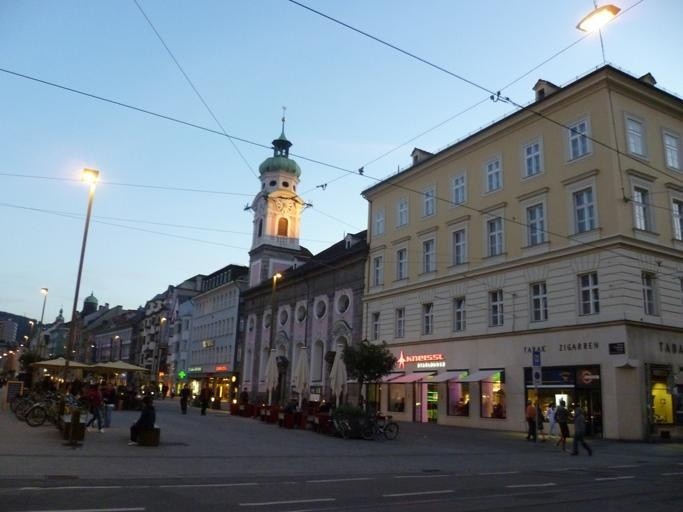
[325,415,352,439]
[358,413,399,441]
[9,381,85,427]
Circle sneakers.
[571,449,593,456]
[128,439,139,446]
[527,436,546,443]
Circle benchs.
[133,419,160,446]
[60,415,85,441]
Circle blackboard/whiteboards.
[6,381,24,404]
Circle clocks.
[275,195,295,214]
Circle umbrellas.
[263,348,280,405]
[29,357,149,385]
[293,346,310,409]
[329,349,347,409]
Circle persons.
[238,387,247,416]
[127,398,154,446]
[524,399,592,457]
[42,376,220,434]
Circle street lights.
[266,272,282,406]
[111,335,120,361]
[0,287,48,362]
[156,318,168,387]
[45,168,101,423]
